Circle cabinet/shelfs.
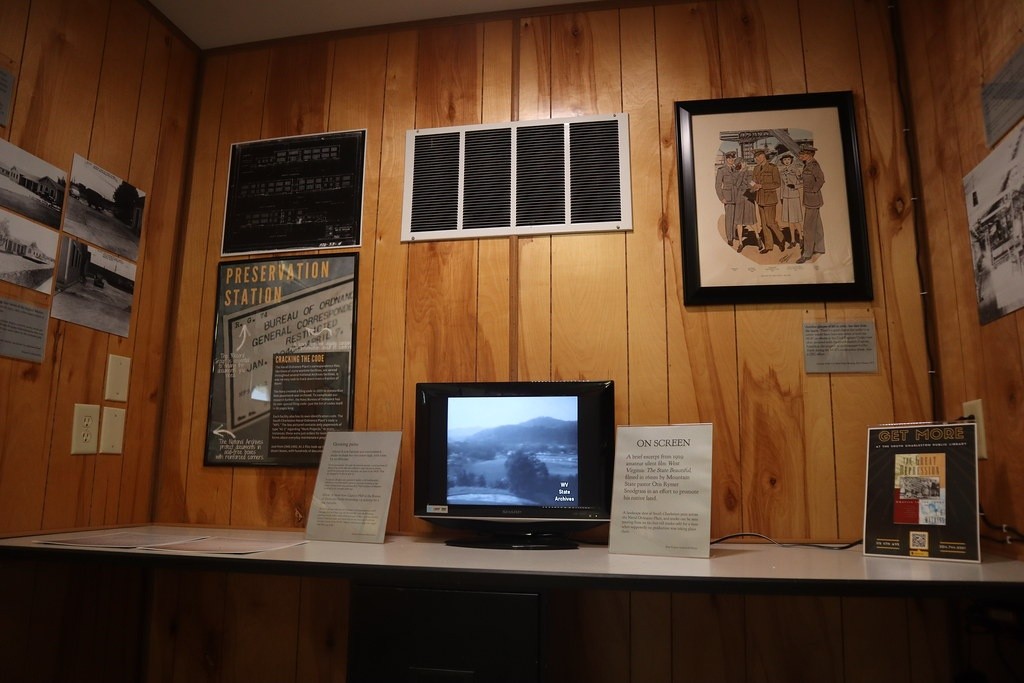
[0,549,1024,683]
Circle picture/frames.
[674,89,874,307]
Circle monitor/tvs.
[411,380,617,549]
